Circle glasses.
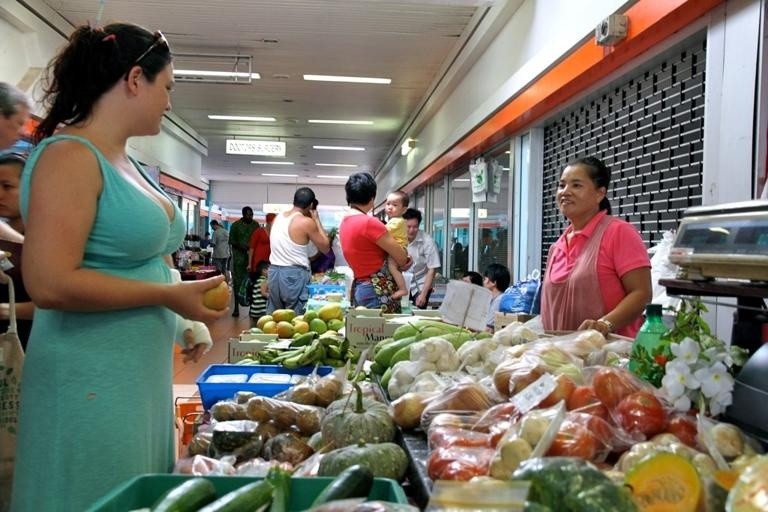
[125,30,172,81]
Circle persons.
[268,186,330,318]
[540,156,652,338]
[0,82,31,156]
[463,264,512,333]
[199,205,280,329]
[10,22,230,512]
[0,152,28,353]
[450,227,508,279]
[340,172,440,316]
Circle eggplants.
[370,321,473,388]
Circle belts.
[268,264,306,270]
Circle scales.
[667,199,768,283]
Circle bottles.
[629,303,670,381]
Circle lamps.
[400,138,416,156]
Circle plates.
[530,400,567,458]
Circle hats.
[266,213,276,223]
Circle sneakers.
[232,310,240,317]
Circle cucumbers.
[128,463,420,512]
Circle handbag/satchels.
[0,274,25,512]
[237,275,254,307]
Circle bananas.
[262,331,360,368]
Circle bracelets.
[597,318,614,331]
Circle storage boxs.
[345,310,440,347]
[196,364,334,408]
[226,333,278,363]
[86,472,411,512]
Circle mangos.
[203,281,230,310]
[257,304,344,338]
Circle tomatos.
[420,353,699,480]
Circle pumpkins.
[319,382,408,484]
[523,448,768,512]
